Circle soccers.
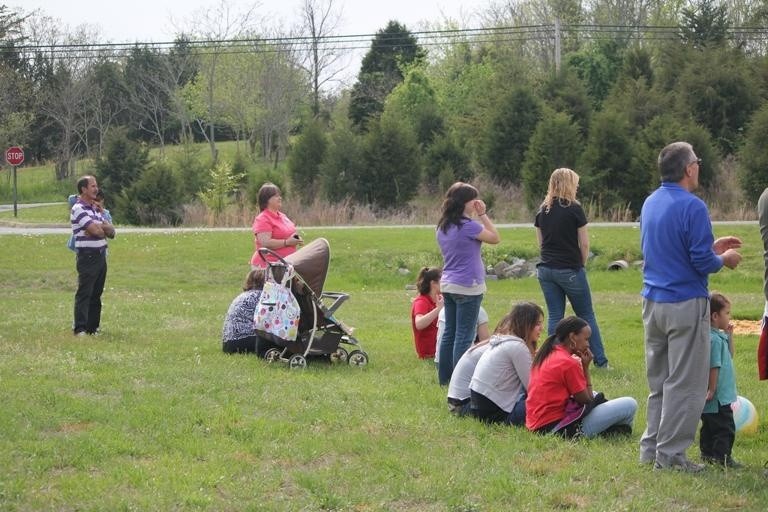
[729,396,758,430]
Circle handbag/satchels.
[254,261,301,342]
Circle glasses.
[690,158,702,165]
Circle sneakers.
[639,457,656,464]
[654,460,706,472]
[700,453,711,464]
[709,456,743,468]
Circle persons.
[469,298,544,421]
[412,266,446,359]
[70,176,116,338]
[534,166,606,368]
[639,140,743,473]
[526,315,639,439]
[67,188,113,250]
[448,315,511,416]
[702,292,744,469]
[434,305,489,377]
[434,182,501,384]
[757,179,768,379]
[250,181,300,272]
[224,268,274,354]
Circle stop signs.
[7,148,24,165]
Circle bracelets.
[477,212,486,219]
[283,238,289,248]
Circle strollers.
[258,238,368,371]
